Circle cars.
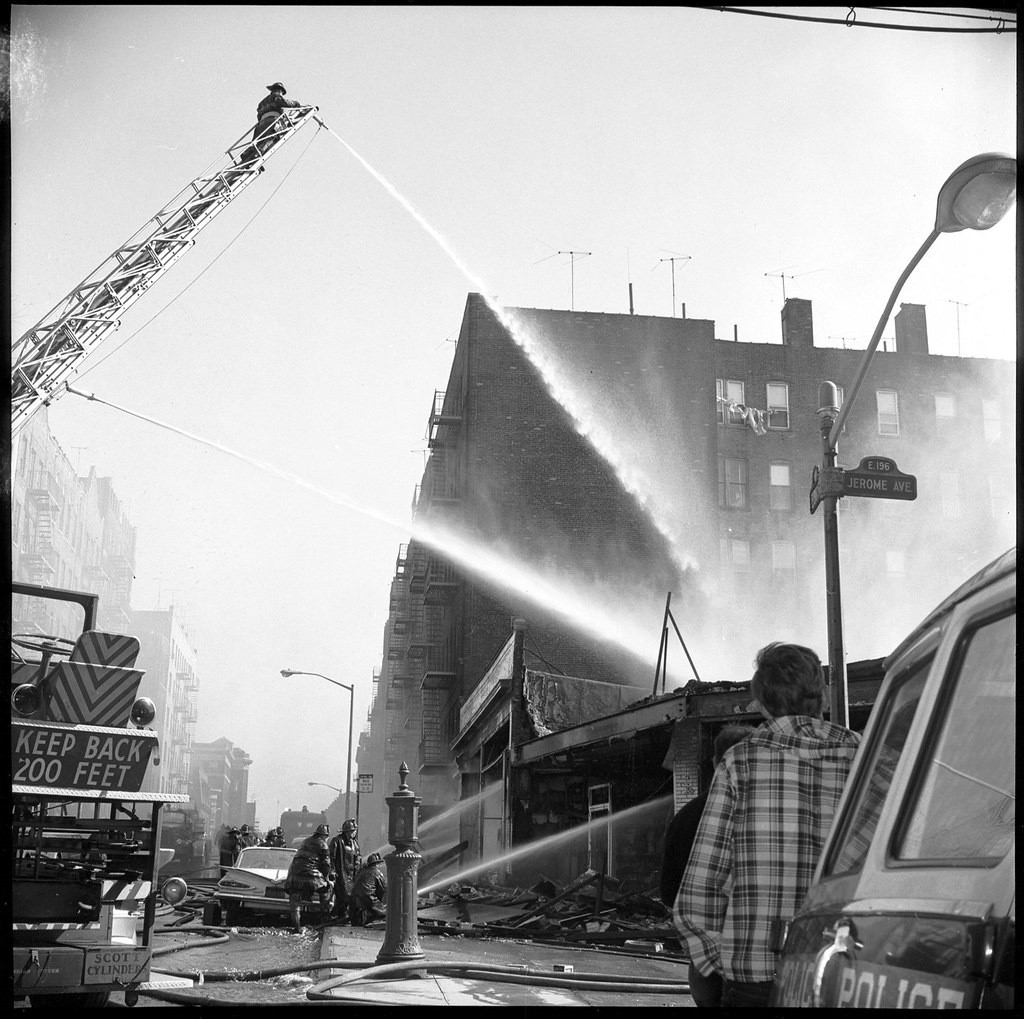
[213,845,336,927]
[769,548,1016,1009]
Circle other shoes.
[289,927,303,934]
[329,908,338,920]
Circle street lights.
[280,668,354,823]
[814,152,1015,727]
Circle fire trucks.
[13,102,328,1019]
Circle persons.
[661,725,759,1005]
[214,818,387,934]
[674,642,902,1008]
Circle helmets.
[276,827,284,837]
[226,827,240,835]
[267,829,278,837]
[367,853,384,866]
[314,824,331,834]
[239,824,251,833]
[338,818,358,831]
